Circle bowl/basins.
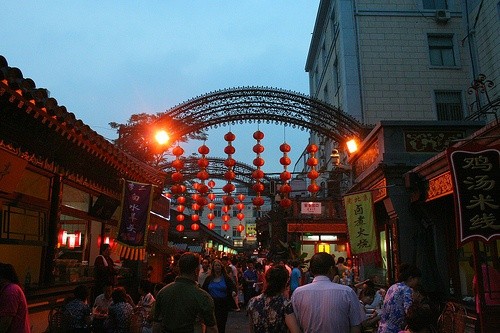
[366,309,375,314]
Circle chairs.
[435,302,467,333]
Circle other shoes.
[234,308,241,311]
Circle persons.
[198,253,314,312]
[470,253,500,314]
[247,266,302,333]
[202,260,233,333]
[0,263,30,333]
[153,253,218,333]
[377,263,429,333]
[290,252,361,333]
[56,244,180,333]
[333,256,383,325]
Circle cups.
[82,261,88,264]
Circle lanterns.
[279,144,292,207]
[237,195,244,232]
[171,146,183,193]
[192,183,200,231]
[164,185,186,231]
[223,132,236,207]
[207,180,215,229]
[197,145,209,206]
[222,205,230,231]
[253,131,264,209]
[308,145,320,193]
